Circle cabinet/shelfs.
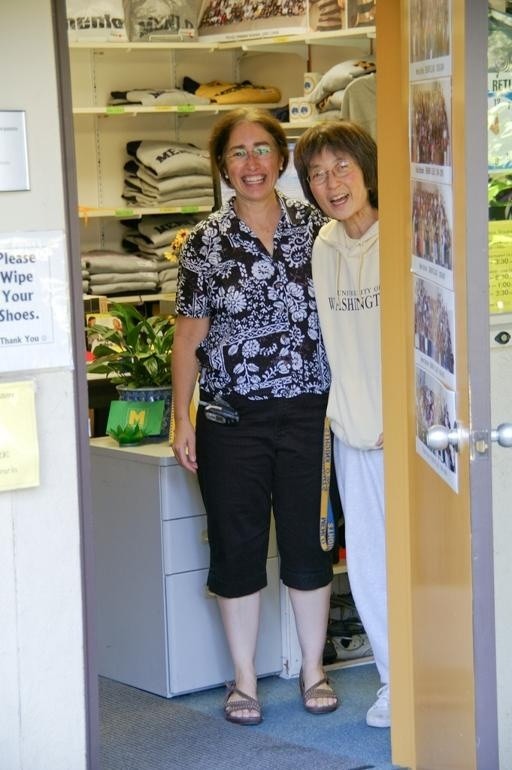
[70,18,379,415]
[89,429,286,697]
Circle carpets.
[96,674,373,770]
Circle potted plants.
[85,301,177,440]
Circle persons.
[292,119,391,731]
[171,107,338,726]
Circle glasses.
[304,158,357,188]
[224,144,280,163]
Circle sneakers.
[367,685,395,729]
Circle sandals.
[222,679,265,724]
[299,668,339,715]
